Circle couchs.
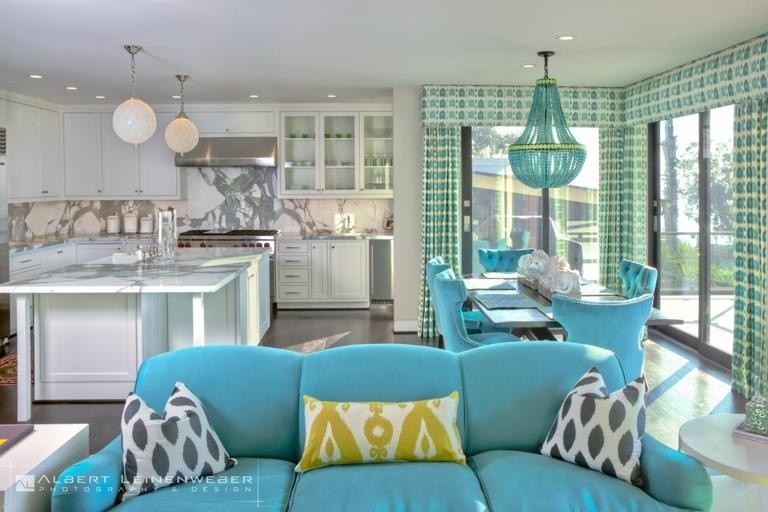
[50,340,713,512]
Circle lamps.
[163,75,200,155]
[111,44,157,146]
[507,51,588,191]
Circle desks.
[0,423,89,512]
[677,413,768,512]
[458,272,627,340]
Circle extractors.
[174,135,278,167]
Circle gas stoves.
[176,228,278,258]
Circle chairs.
[477,248,536,272]
[550,293,654,383]
[433,267,522,353]
[618,258,658,300]
[425,256,513,334]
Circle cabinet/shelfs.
[275,236,310,303]
[7,99,63,204]
[63,111,188,201]
[275,101,393,200]
[76,243,123,263]
[310,240,370,302]
[9,246,72,335]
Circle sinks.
[303,233,367,240]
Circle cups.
[285,132,317,138]
[321,130,358,141]
[325,158,360,167]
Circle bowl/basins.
[285,159,315,166]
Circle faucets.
[347,216,354,232]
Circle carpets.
[0,343,34,384]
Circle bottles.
[122,211,139,235]
[139,215,154,235]
[131,236,177,263]
[105,213,121,234]
[363,153,393,168]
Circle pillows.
[294,390,466,474]
[115,381,239,504]
[537,365,649,491]
